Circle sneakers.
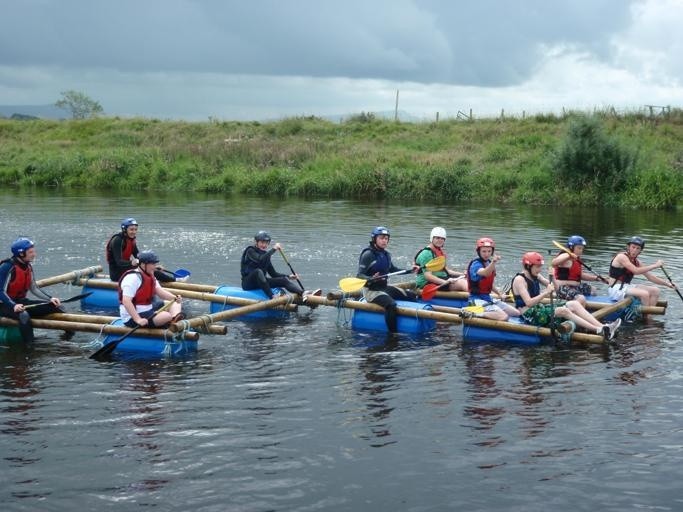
[607,318,622,339]
[302,289,322,307]
[600,326,611,341]
[461,309,472,319]
[171,313,186,332]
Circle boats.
[302,279,668,346]
[0,266,302,356]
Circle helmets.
[254,231,270,241]
[523,251,544,269]
[476,238,495,257]
[11,237,33,253]
[139,250,159,263]
[371,226,390,240]
[627,236,644,249]
[122,218,138,226]
[568,235,586,247]
[430,226,447,243]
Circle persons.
[460,237,520,322]
[117,251,187,327]
[511,251,622,340]
[240,231,322,300]
[0,237,76,343]
[551,236,609,309]
[411,226,468,293]
[106,218,176,282]
[357,226,421,340]
[607,236,676,326]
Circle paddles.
[89,295,178,361]
[551,240,604,280]
[421,275,466,301]
[22,291,93,310]
[339,256,446,292]
[462,290,515,317]
[136,259,191,282]
